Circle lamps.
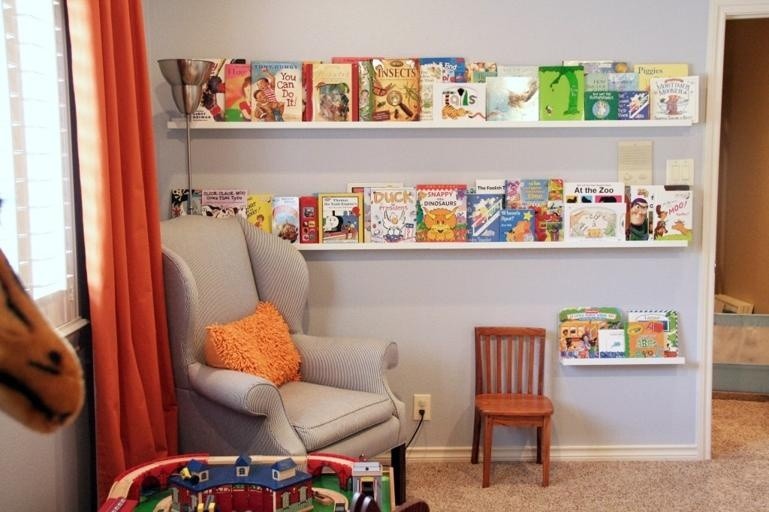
[157,58,218,214]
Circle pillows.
[203,301,304,389]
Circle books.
[558,306,678,357]
[170,179,694,245]
[180,56,700,125]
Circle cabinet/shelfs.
[165,118,694,366]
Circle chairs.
[471,326,554,488]
[160,213,406,506]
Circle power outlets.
[412,394,432,422]
